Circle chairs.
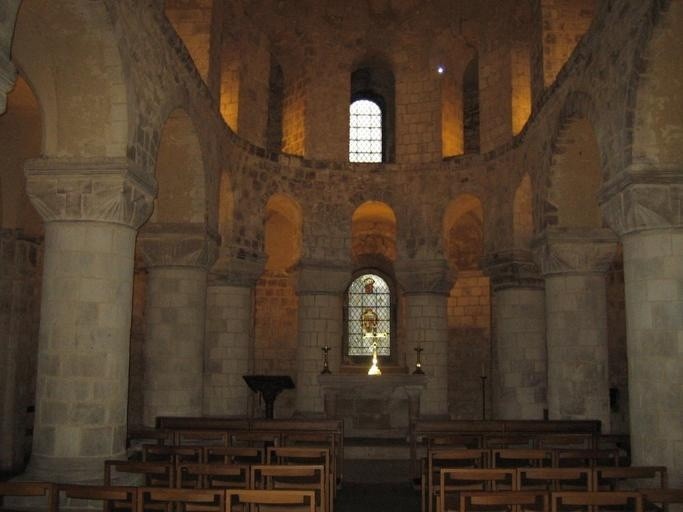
[404,417,682,510]
[1,414,344,510]
